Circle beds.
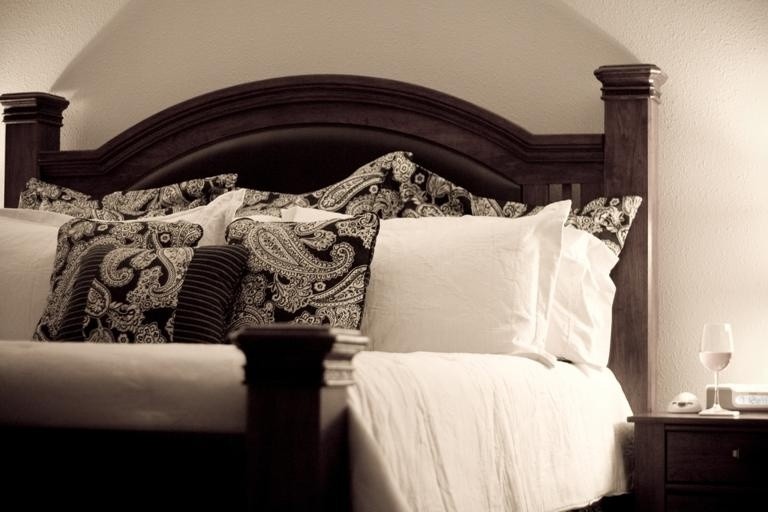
[0,61,669,512]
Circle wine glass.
[698,321,733,416]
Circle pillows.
[0,151,644,367]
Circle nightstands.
[627,412,768,512]
